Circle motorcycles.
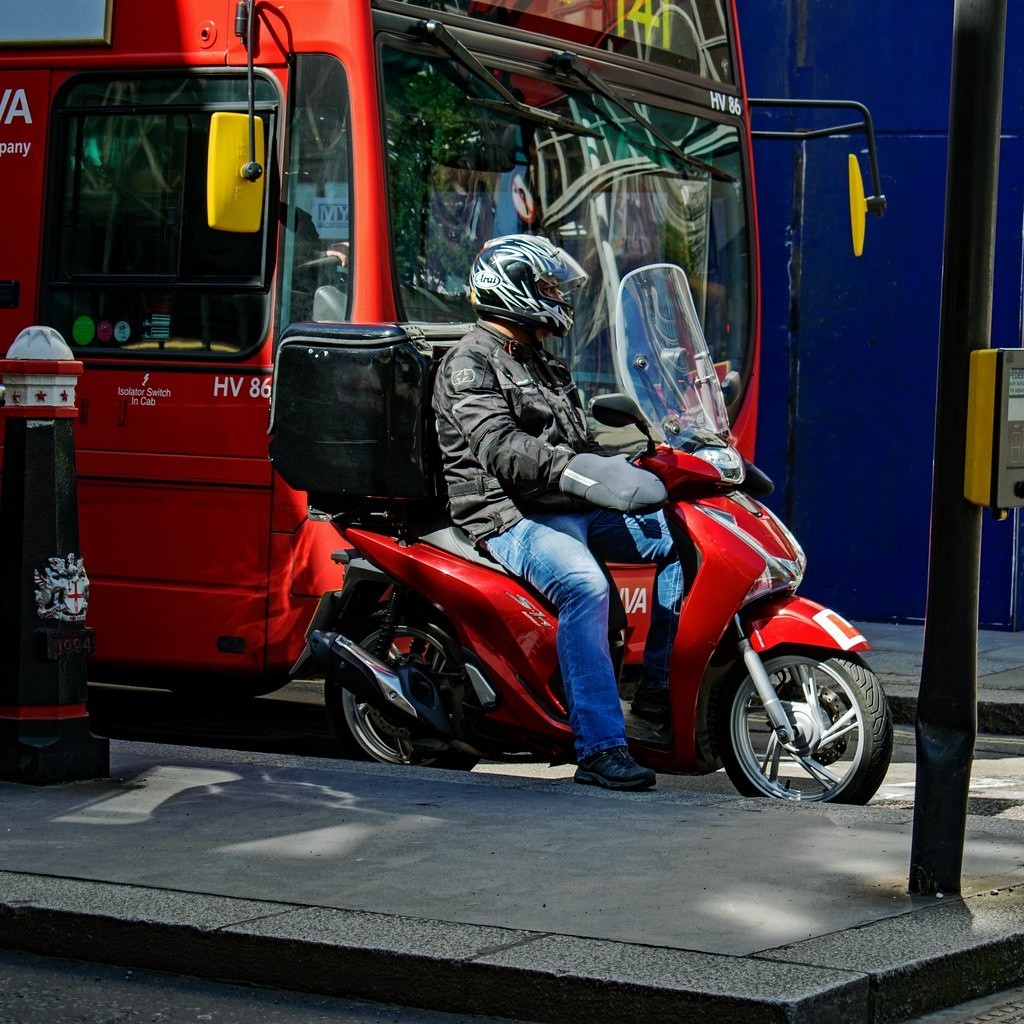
[267,259,892,807]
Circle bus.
[0,0,888,700]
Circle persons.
[226,153,351,357]
[431,233,700,790]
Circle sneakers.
[574,745,657,791]
[631,683,671,718]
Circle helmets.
[469,234,589,338]
